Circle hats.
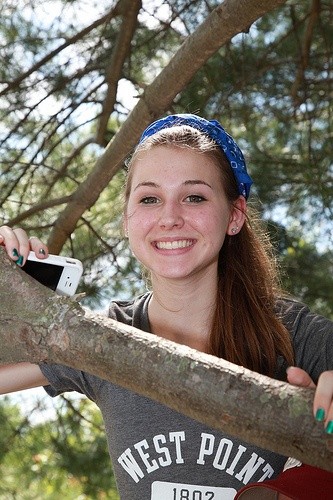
[233,466,333,500]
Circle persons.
[0,114,333,500]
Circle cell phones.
[16,250,83,297]
